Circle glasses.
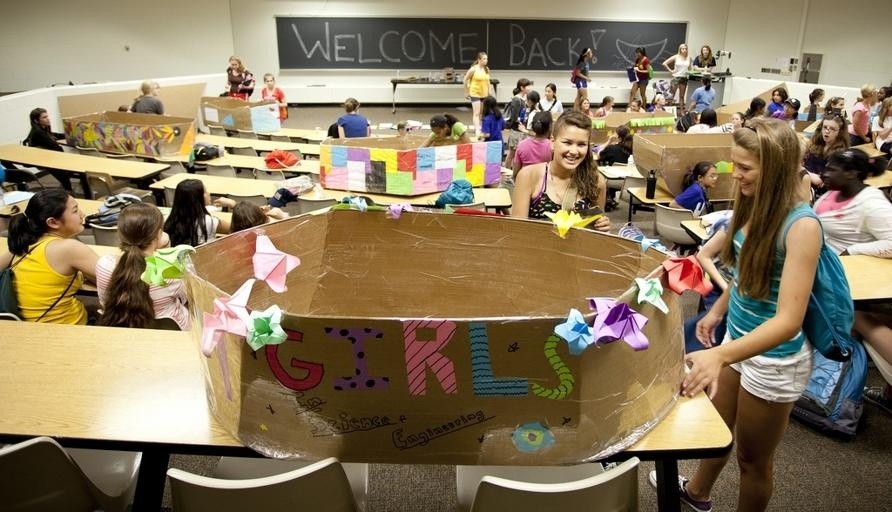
[825,110,845,128]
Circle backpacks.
[642,56,653,80]
[1,241,82,321]
[788,321,866,439]
[776,209,854,365]
[571,64,583,84]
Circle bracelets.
[282,213,290,220]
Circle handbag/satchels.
[501,97,521,129]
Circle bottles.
[627,154,636,175]
[395,69,401,80]
[647,170,657,200]
[428,71,441,81]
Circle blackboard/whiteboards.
[273,15,689,78]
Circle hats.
[783,98,801,110]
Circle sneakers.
[648,466,714,511]
[604,198,620,212]
[861,382,891,414]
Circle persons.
[0,189,98,325]
[477,77,633,233]
[230,201,286,234]
[164,179,236,245]
[627,46,650,108]
[695,220,735,346]
[669,161,718,218]
[130,81,163,116]
[397,123,413,137]
[648,43,748,133]
[95,203,195,330]
[627,99,645,113]
[464,52,497,138]
[262,73,287,126]
[649,118,825,512]
[25,108,81,192]
[745,85,892,195]
[854,310,892,415]
[573,47,593,112]
[338,97,371,139]
[226,58,256,103]
[420,113,470,147]
[119,104,130,112]
[813,149,892,261]
